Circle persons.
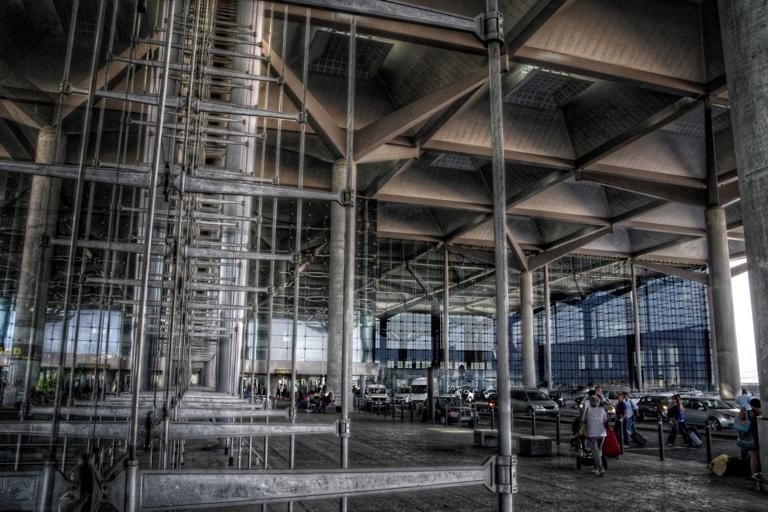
[295,380,335,415]
[665,394,693,448]
[454,387,461,400]
[740,398,763,482]
[352,385,368,399]
[275,383,287,401]
[253,379,267,396]
[578,384,640,477]
[39,376,102,401]
[736,388,752,411]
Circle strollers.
[572,424,608,470]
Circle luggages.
[682,422,703,448]
[603,425,621,460]
[627,431,647,447]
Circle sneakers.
[591,468,606,477]
[751,472,763,481]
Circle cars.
[415,388,566,425]
[636,393,741,432]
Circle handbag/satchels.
[732,418,756,448]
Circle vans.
[366,376,438,408]
